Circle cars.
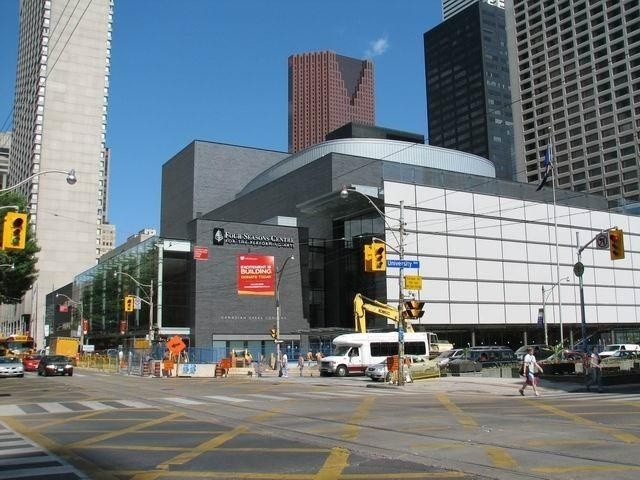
[0,348,46,378]
[37,355,76,377]
[514,341,640,379]
[432,346,466,369]
[230,347,252,367]
[365,351,438,383]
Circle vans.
[459,344,517,369]
[463,349,516,367]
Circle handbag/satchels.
[518,365,529,377]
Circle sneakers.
[519,390,539,396]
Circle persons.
[586,346,603,392]
[243,350,323,378]
[519,347,544,396]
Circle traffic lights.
[372,242,386,272]
[270,328,277,339]
[415,301,427,318]
[404,299,416,318]
[2,211,29,252]
[124,296,134,311]
[607,229,625,261]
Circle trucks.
[315,330,433,378]
[46,336,80,359]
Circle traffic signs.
[386,260,421,269]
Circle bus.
[0,334,38,362]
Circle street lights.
[56,294,86,352]
[540,276,572,345]
[338,187,407,388]
[0,262,16,270]
[0,167,80,198]
[275,253,296,372]
[112,269,154,349]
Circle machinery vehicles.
[351,291,456,357]
